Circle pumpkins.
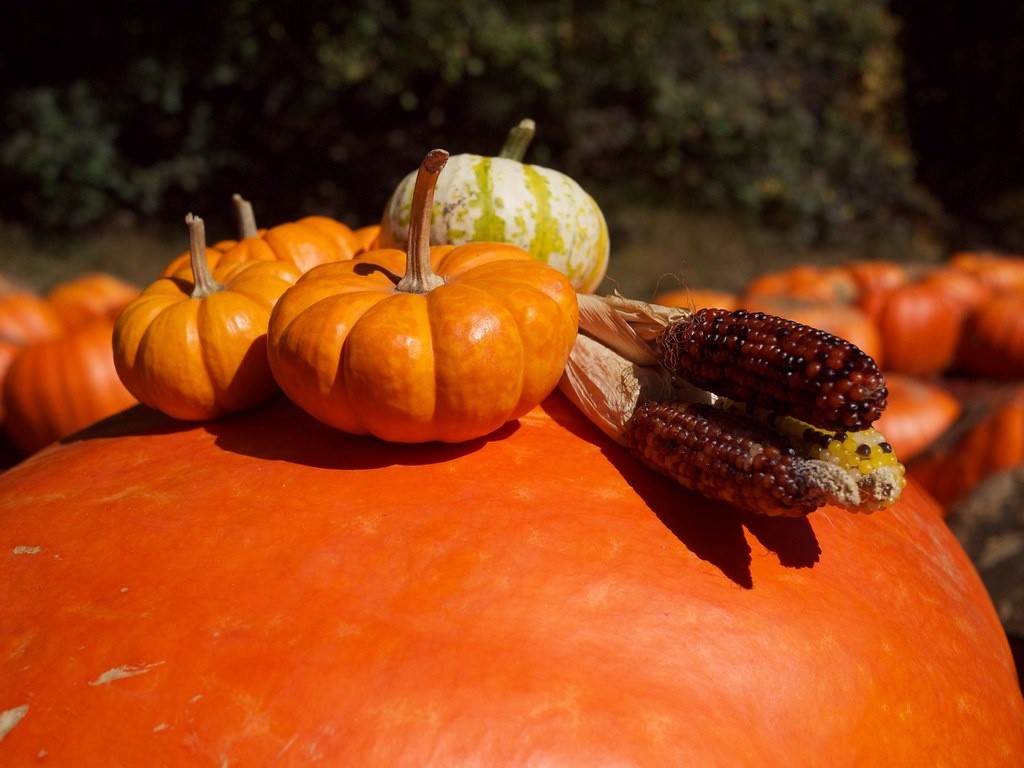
[0,383,1024,768]
[0,119,609,459]
[652,248,1024,521]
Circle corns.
[626,307,909,519]
